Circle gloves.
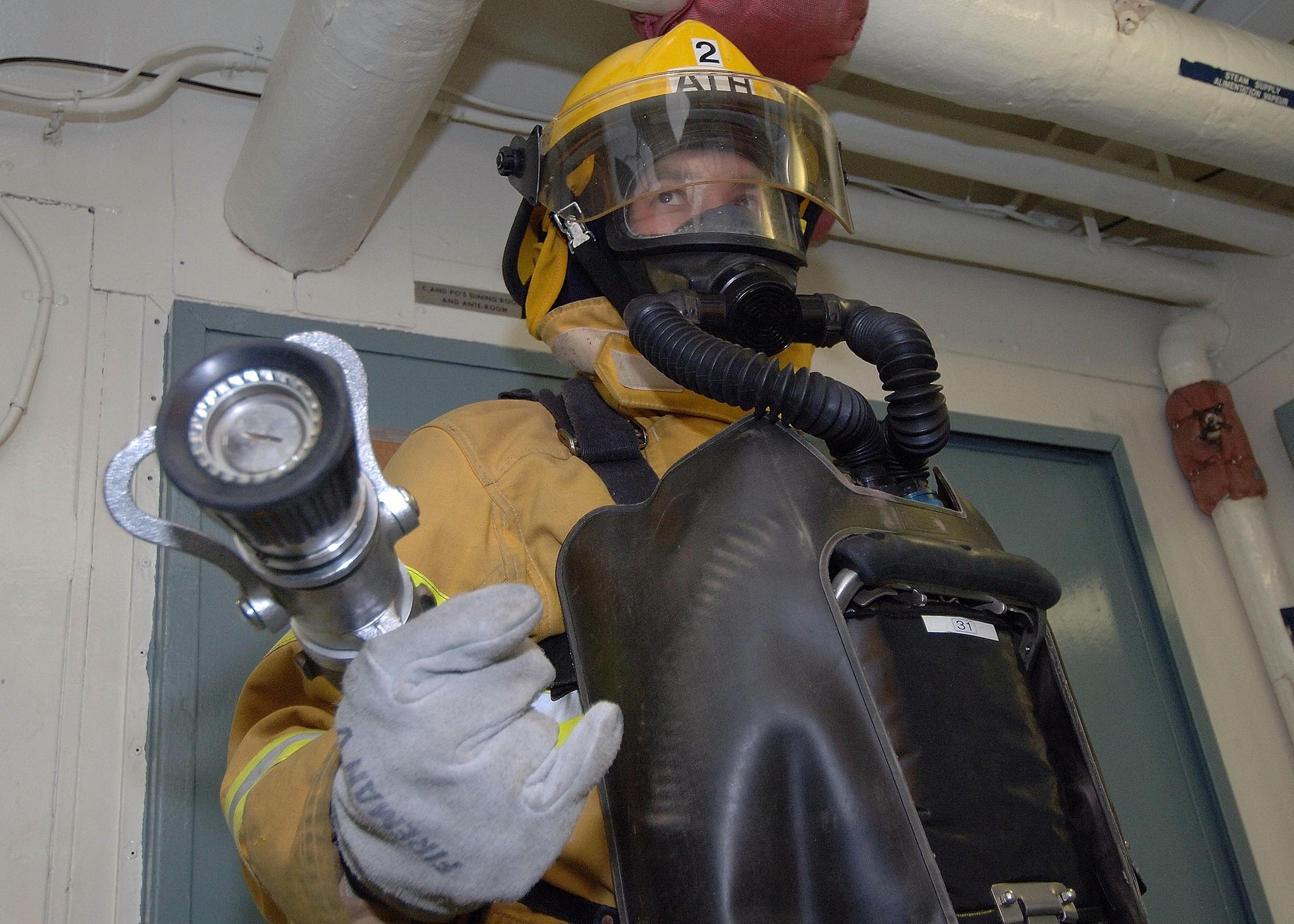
[327,578,623,920]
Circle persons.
[211,19,1150,924]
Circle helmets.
[497,18,853,345]
